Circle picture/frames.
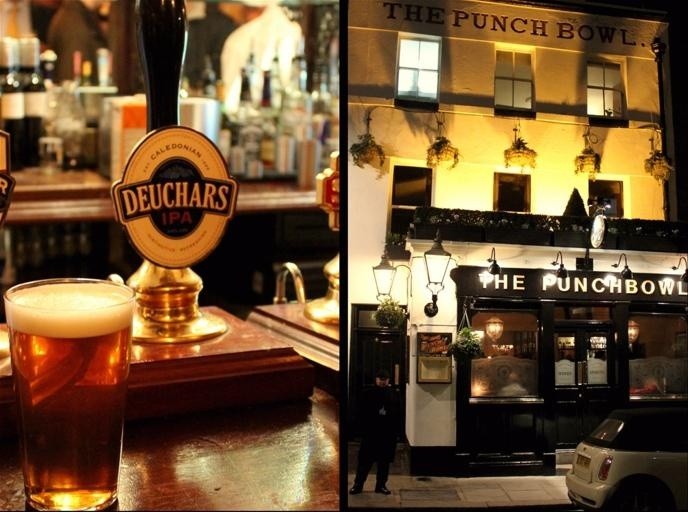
[417,331,451,383]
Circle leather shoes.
[350,486,363,494]
[375,486,390,495]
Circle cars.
[562,405,684,508]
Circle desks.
[6,164,323,321]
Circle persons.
[350,369,400,496]
[0,0,307,94]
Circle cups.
[4,277,136,511]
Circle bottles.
[194,27,337,178]
[0,33,114,173]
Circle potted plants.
[645,151,673,186]
[447,328,481,364]
[504,138,537,175]
[349,134,385,180]
[372,300,405,333]
[427,136,458,169]
[575,146,601,180]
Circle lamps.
[672,257,688,285]
[553,251,570,282]
[614,252,636,281]
[488,248,502,278]
[628,319,640,344]
[423,229,452,316]
[484,316,504,341]
[373,255,413,310]
